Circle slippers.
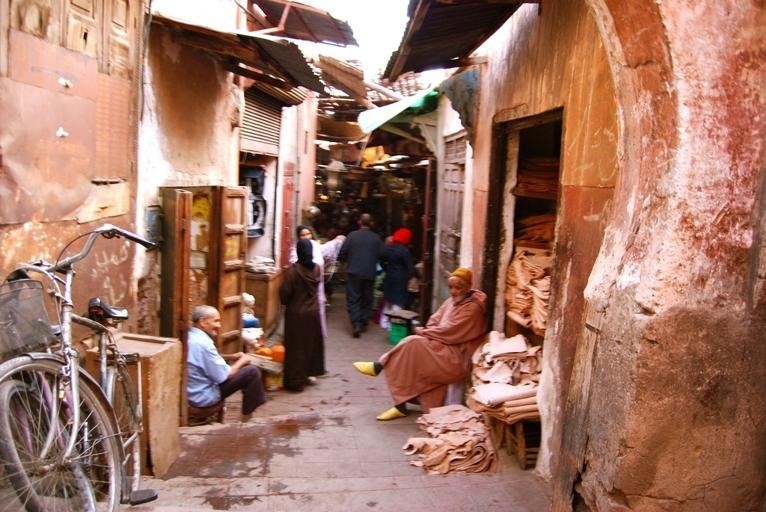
[353,361,377,376]
[376,406,407,420]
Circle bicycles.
[0,224,157,512]
[0,260,126,512]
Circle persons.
[353,268,487,421]
[280,239,323,392]
[289,226,328,340]
[338,213,383,338]
[379,228,422,331]
[184,305,267,423]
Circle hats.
[451,268,473,286]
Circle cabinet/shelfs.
[246,268,280,337]
[87,334,180,478]
